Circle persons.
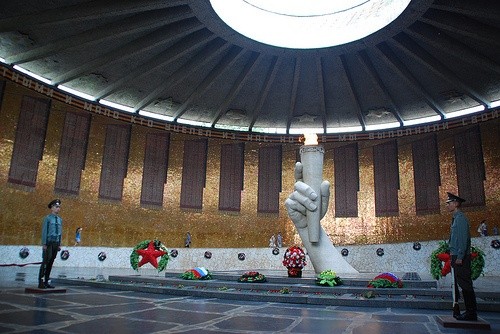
[277,231,283,248]
[185,232,191,248]
[446,192,478,321]
[74,227,83,246]
[285,162,358,275]
[37,199,63,289]
[478,220,498,237]
[269,234,276,248]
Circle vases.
[316,277,342,286]
[243,279,266,282]
[287,266,305,278]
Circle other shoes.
[38,283,47,289]
[45,283,55,288]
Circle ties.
[449,217,455,243]
[55,217,58,234]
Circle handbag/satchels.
[477,228,482,232]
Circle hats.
[48,199,61,208]
[446,192,466,203]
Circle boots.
[454,291,477,321]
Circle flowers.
[373,270,403,289]
[319,271,340,280]
[239,270,264,281]
[284,246,307,268]
